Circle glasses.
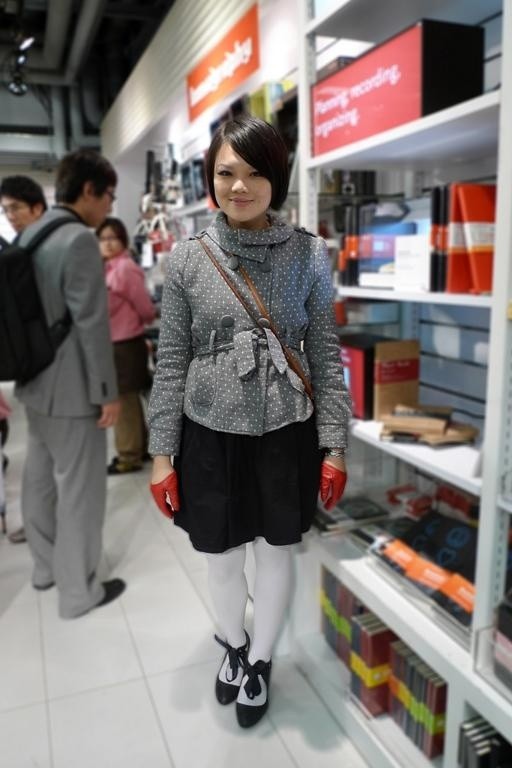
[106,190,118,205]
[0,203,29,215]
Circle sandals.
[112,453,151,464]
[107,463,143,476]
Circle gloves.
[320,462,347,511]
[150,470,181,519]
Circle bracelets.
[321,444,350,459]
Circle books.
[336,179,496,294]
[349,613,511,768]
[372,336,423,421]
[379,404,479,449]
[311,484,512,691]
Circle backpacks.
[0,216,88,389]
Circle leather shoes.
[7,527,27,544]
[235,655,272,728]
[88,578,126,609]
[214,628,250,705]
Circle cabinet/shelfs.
[290,2,510,766]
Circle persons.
[96,218,156,476]
[0,388,16,473]
[0,173,49,545]
[143,118,350,730]
[0,147,126,620]
